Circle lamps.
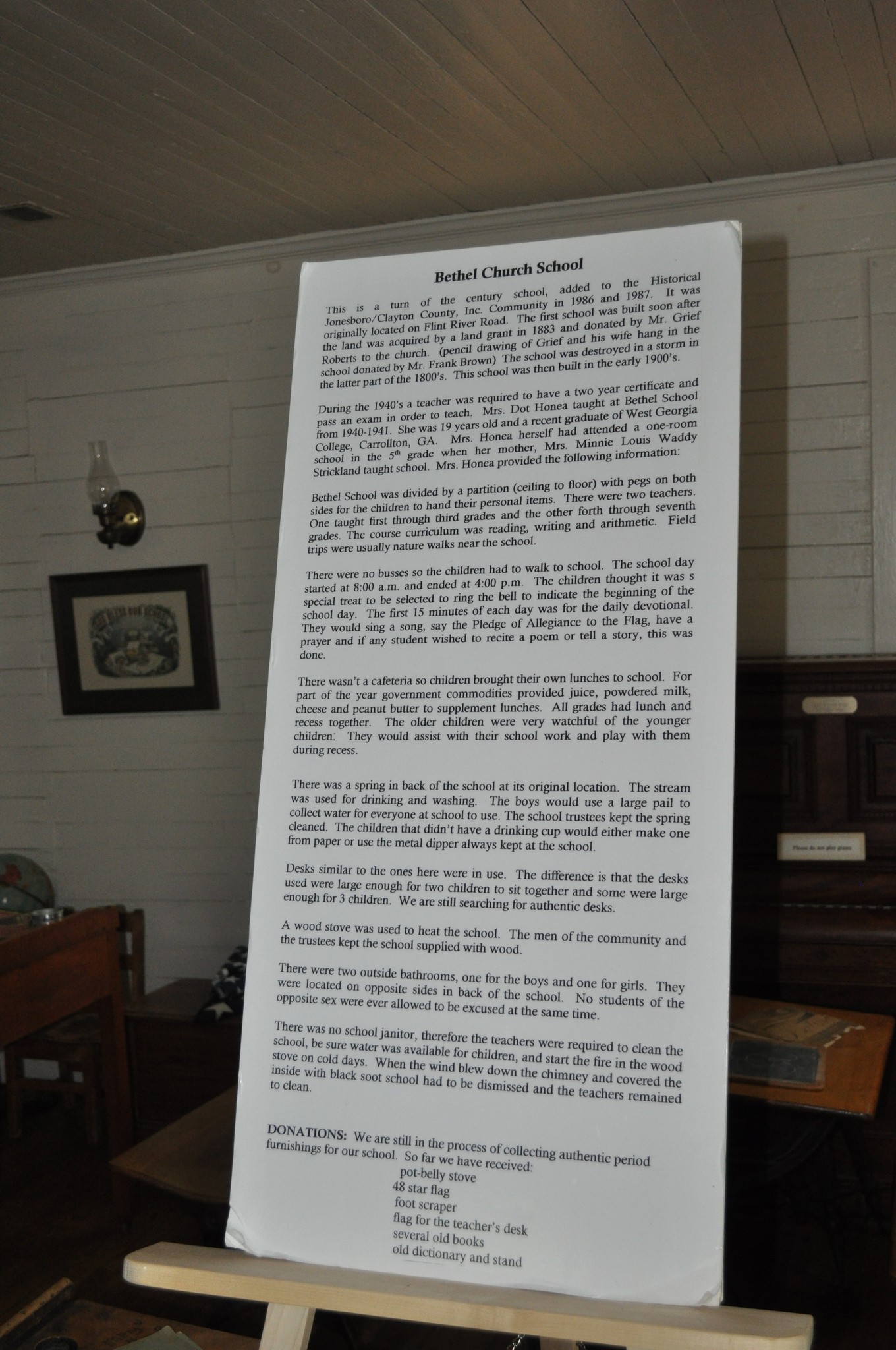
[80,438,146,551]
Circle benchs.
[123,1240,813,1350]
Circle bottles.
[29,907,64,928]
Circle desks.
[729,995,893,1307]
[1,897,141,1232]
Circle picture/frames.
[48,565,219,715]
